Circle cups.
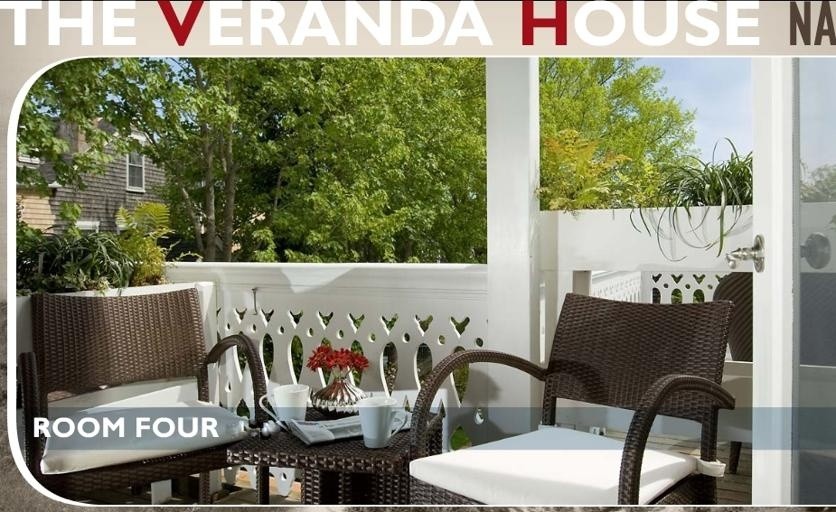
[355,397,407,448]
[258,385,310,425]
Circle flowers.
[306,344,370,380]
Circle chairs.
[407,291,738,505]
[20,286,270,505]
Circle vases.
[312,378,367,418]
